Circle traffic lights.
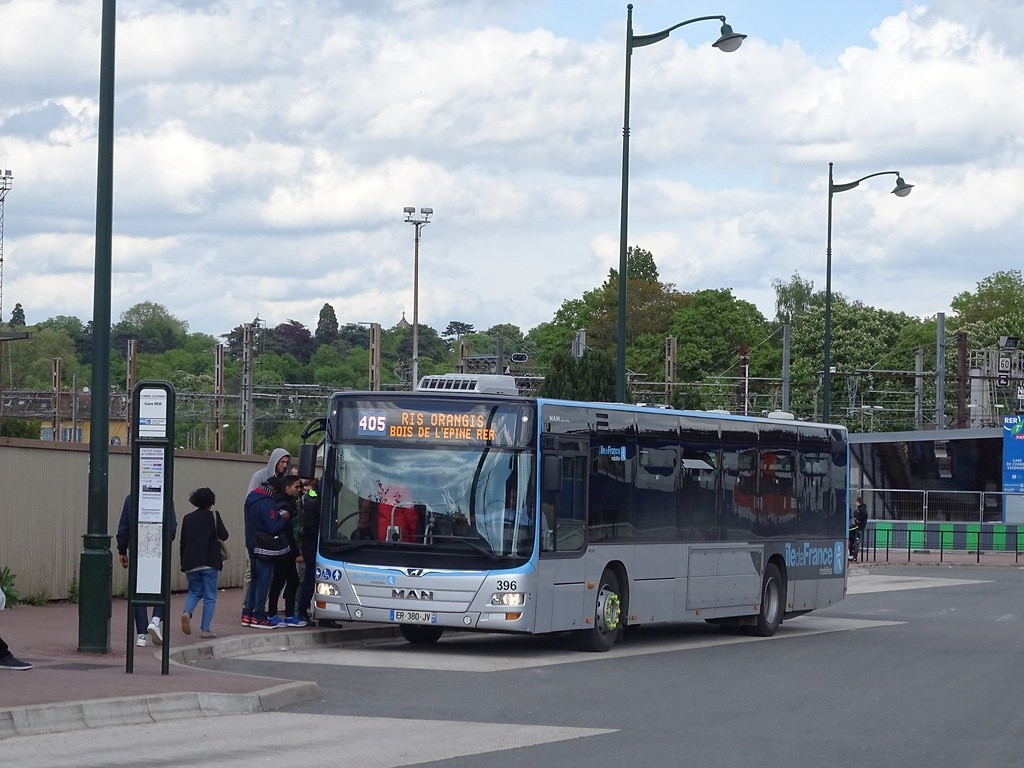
[511,352,528,363]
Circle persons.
[359,463,418,542]
[240,476,290,628]
[477,487,550,537]
[180,488,229,638]
[267,466,342,628]
[117,494,178,646]
[0,638,33,669]
[849,497,868,560]
[241,448,292,609]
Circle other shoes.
[848,556,856,561]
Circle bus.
[296,374,851,653]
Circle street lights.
[823,159,914,421]
[404,205,433,390]
[613,4,747,402]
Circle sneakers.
[181,614,192,635]
[241,614,251,626]
[250,617,276,629]
[203,630,216,638]
[136,636,150,647]
[285,617,307,627]
[1,652,33,670]
[299,614,315,626]
[319,620,343,629]
[148,622,162,645]
[269,615,284,627]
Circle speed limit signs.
[999,357,1011,372]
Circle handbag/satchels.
[217,539,231,562]
